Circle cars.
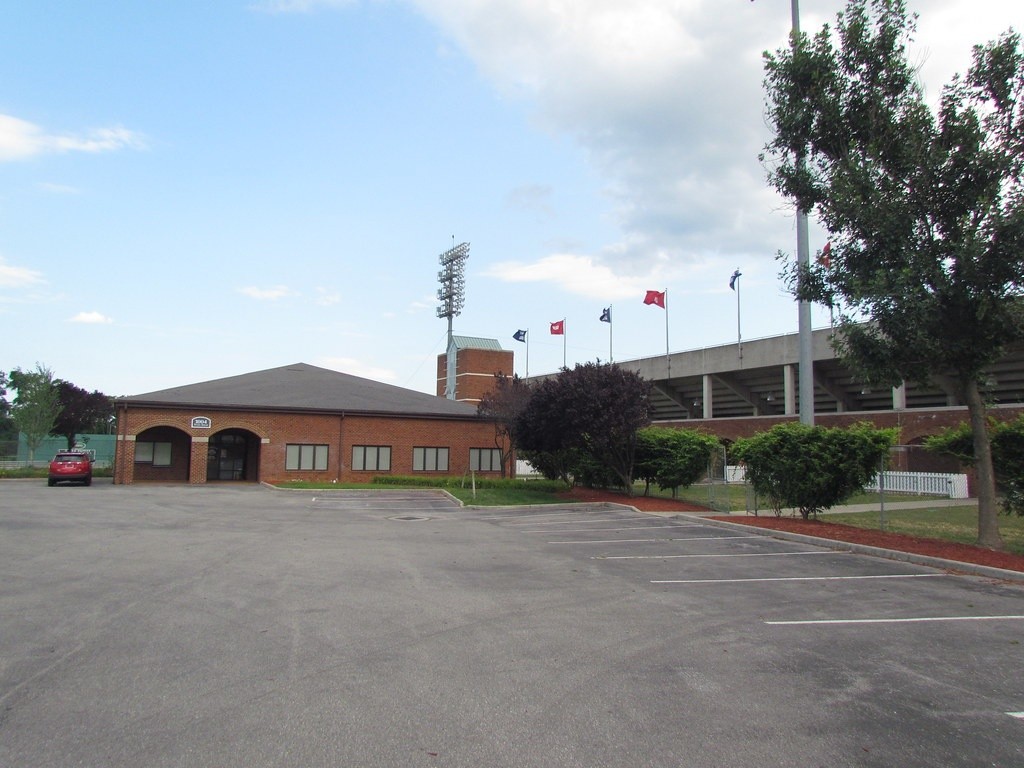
[48,452,96,486]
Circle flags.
[551,320,563,334]
[513,330,526,343]
[729,269,743,291]
[643,290,666,308]
[600,307,611,322]
[817,242,831,270]
